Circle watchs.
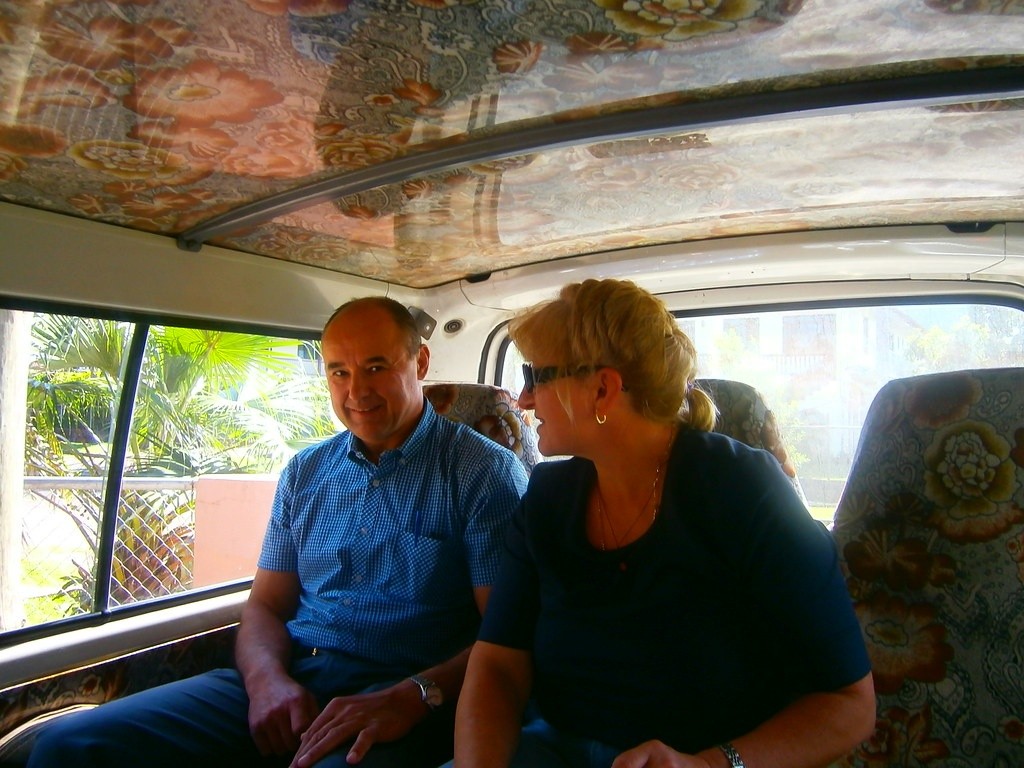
[408,673,444,712]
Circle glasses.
[522,362,628,393]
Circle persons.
[24,297,526,768]
[440,278,877,768]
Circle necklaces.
[601,427,674,552]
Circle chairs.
[422,383,544,479]
[692,379,809,509]
[826,366,1023,767]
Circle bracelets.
[719,742,746,768]
